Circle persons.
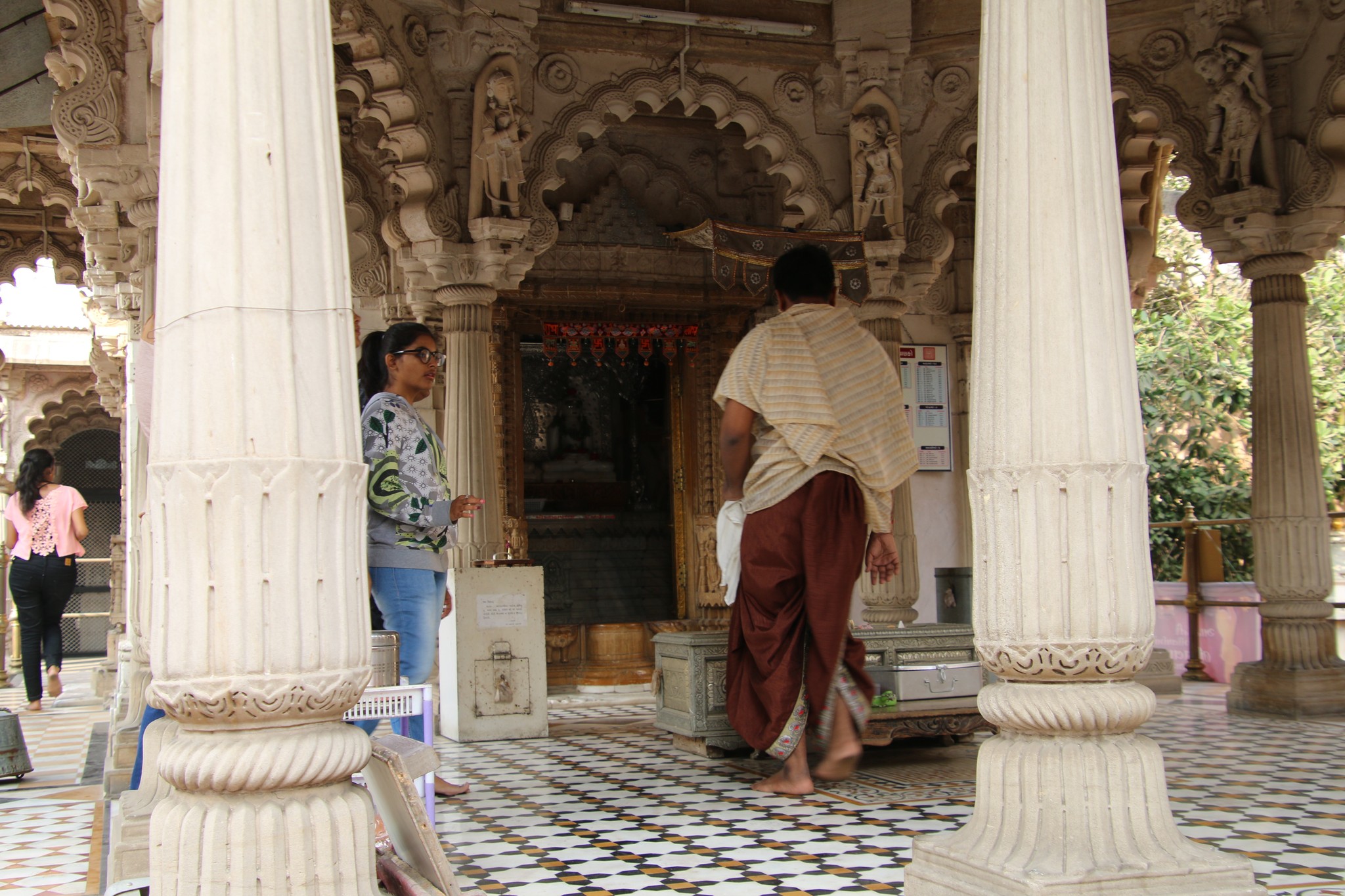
[850,115,903,240]
[346,322,488,796]
[44,49,102,206]
[714,242,920,796]
[474,67,529,219]
[6,448,88,711]
[0,402,9,424]
[1194,37,1272,195]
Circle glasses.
[392,348,446,367]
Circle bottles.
[508,540,514,559]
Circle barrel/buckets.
[368,631,399,688]
[1,709,34,781]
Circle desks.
[651,620,995,761]
